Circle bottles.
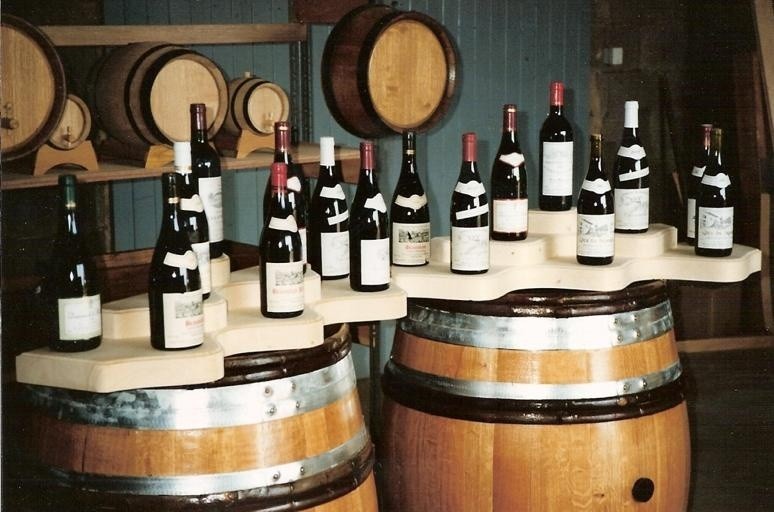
[148,171,205,351]
[612,100,652,234]
[577,132,616,267]
[538,81,574,211]
[172,140,213,300]
[187,102,225,259]
[44,172,102,352]
[490,103,530,241]
[694,124,736,257]
[449,132,492,275]
[686,124,713,246]
[258,120,432,320]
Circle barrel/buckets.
[84,43,228,149]
[29,323,379,512]
[48,94,91,151]
[220,77,289,137]
[382,281,692,512]
[0,11,67,162]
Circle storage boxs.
[669,284,743,339]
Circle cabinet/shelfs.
[0,22,381,442]
